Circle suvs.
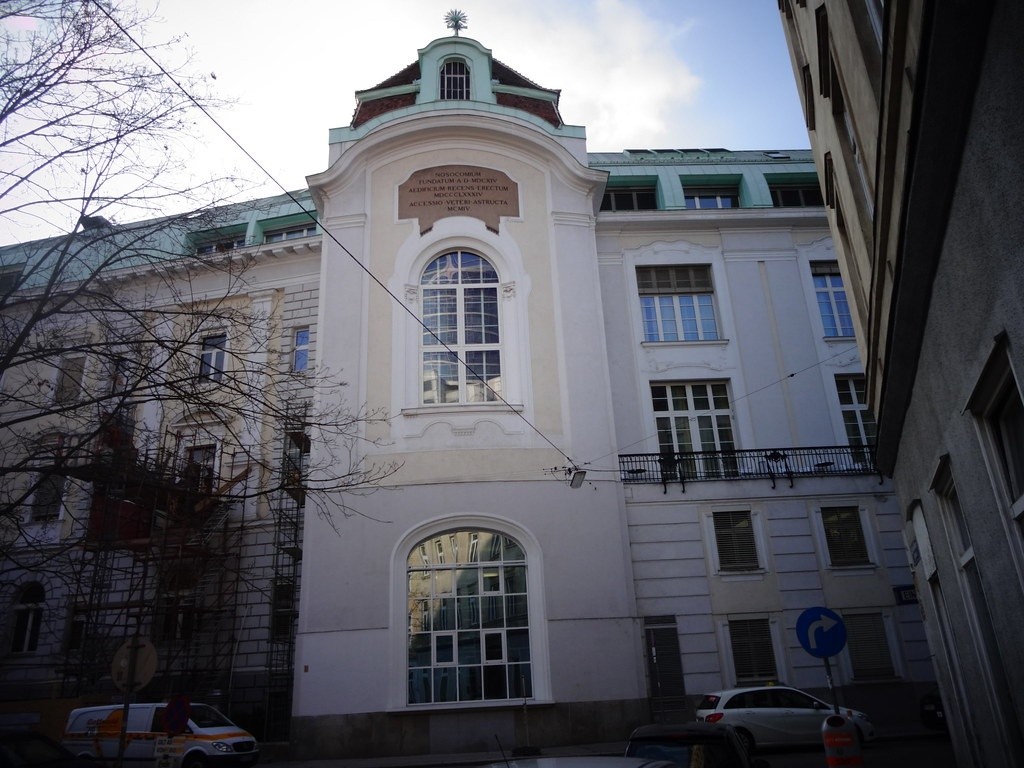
[695,686,876,754]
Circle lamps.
[564,468,587,489]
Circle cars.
[467,754,681,768]
[623,719,774,768]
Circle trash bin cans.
[820,713,864,768]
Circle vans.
[58,702,263,768]
